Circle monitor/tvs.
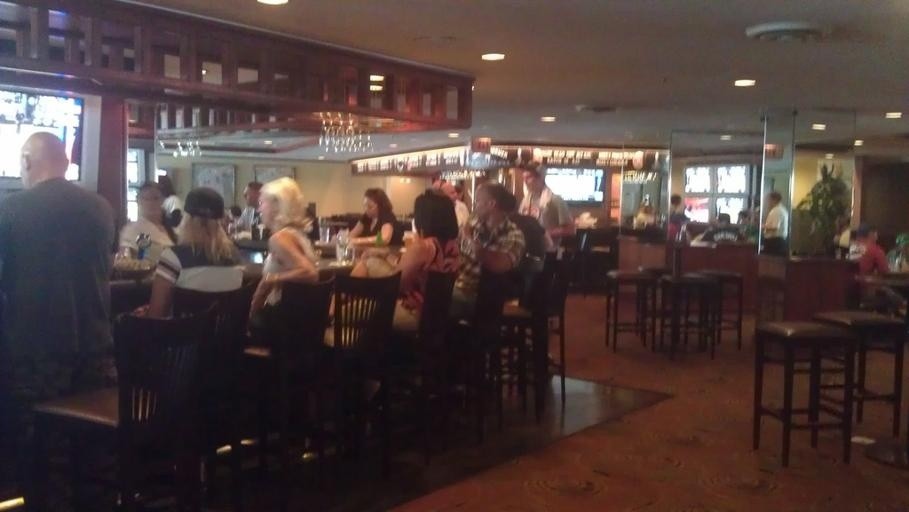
[0,82,104,196]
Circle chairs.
[753,310,905,464]
[31,252,574,511]
[606,267,743,360]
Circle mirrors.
[790,108,856,258]
[759,109,794,258]
[621,151,672,239]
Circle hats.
[184,186,224,218]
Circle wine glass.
[318,135,374,153]
[336,228,350,267]
[171,140,202,159]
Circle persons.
[838,220,909,299]
[1,132,577,454]
[633,189,788,260]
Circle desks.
[855,273,909,354]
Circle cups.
[336,248,354,267]
[319,227,330,244]
